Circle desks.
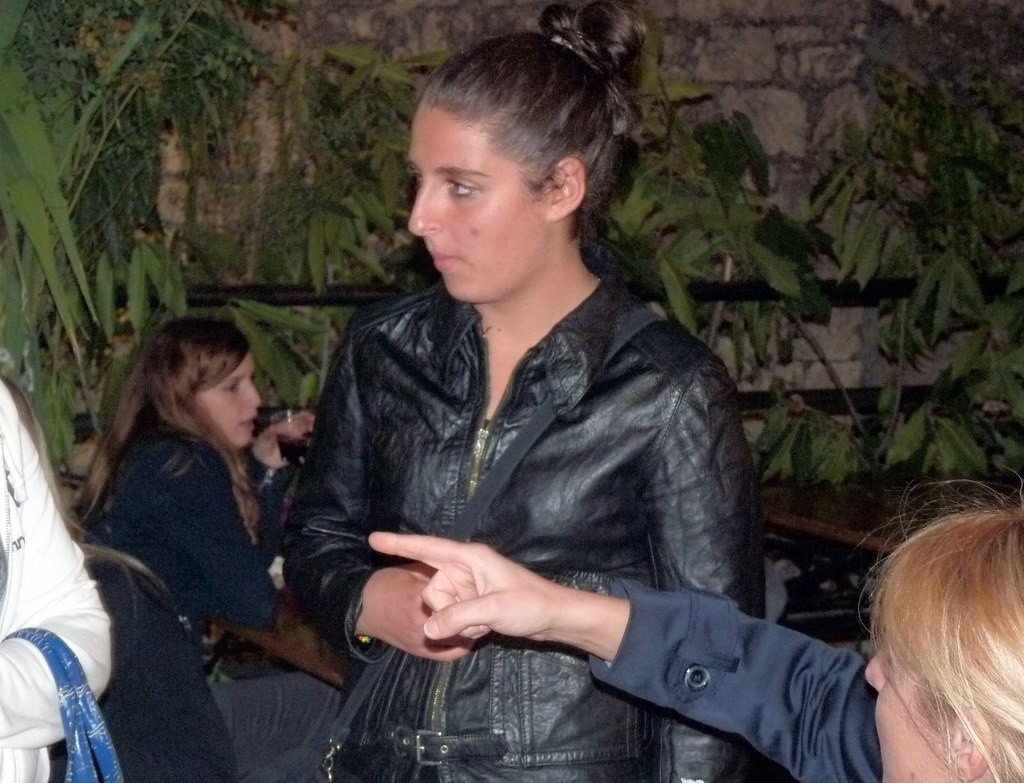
[211,590,346,688]
[750,466,1024,558]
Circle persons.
[1,380,116,783]
[70,317,345,783]
[366,511,1024,781]
[282,0,765,782]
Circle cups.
[270,408,312,464]
